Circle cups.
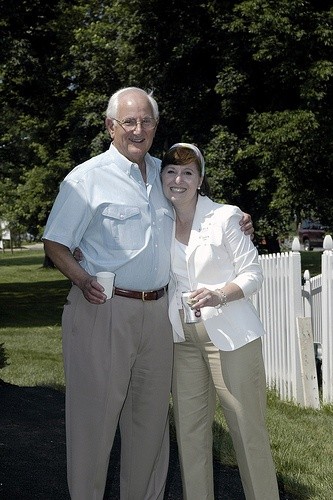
[96,272,115,299]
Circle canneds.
[181,290,201,324]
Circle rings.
[205,297,209,302]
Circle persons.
[41,87,254,500]
[74,143,279,500]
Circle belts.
[114,284,168,302]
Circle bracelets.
[215,289,229,309]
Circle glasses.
[112,117,158,131]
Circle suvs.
[298,219,327,251]
